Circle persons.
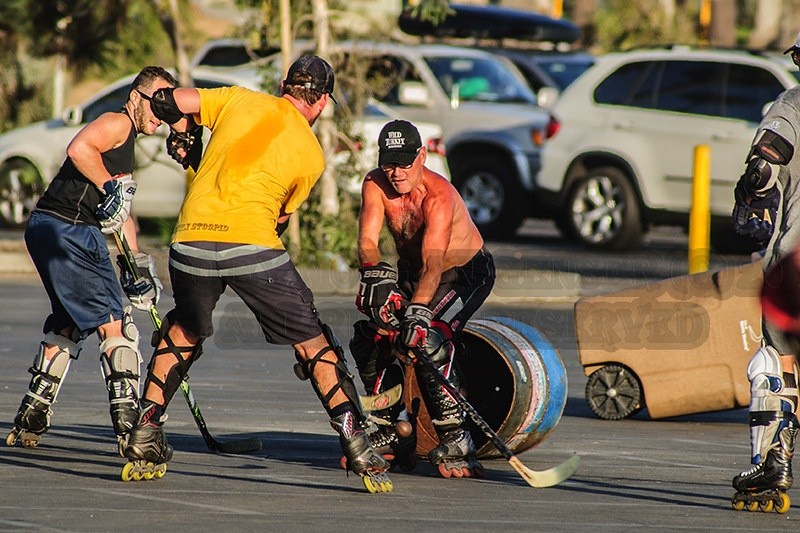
[120,55,394,493]
[341,118,498,480]
[5,66,182,457]
[732,34,800,515]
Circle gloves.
[356,261,402,325]
[96,174,137,235]
[396,304,432,349]
[730,172,781,250]
[118,252,163,311]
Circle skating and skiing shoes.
[106,375,140,455]
[121,413,174,482]
[430,420,488,478]
[331,410,403,492]
[731,446,795,514]
[340,414,400,474]
[7,372,61,446]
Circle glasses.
[380,162,414,172]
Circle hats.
[378,120,422,166]
[286,55,339,105]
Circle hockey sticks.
[190,156,403,410]
[416,348,579,488]
[108,223,264,456]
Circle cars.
[535,43,799,252]
[1,66,454,230]
[192,4,602,240]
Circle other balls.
[396,422,413,439]
